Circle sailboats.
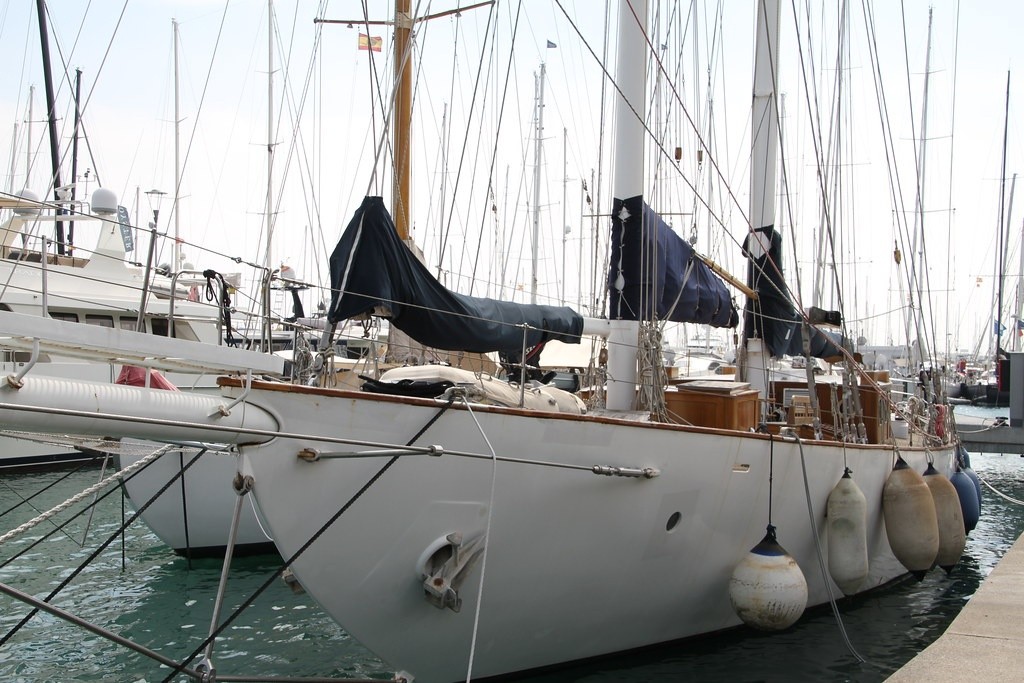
[0,0,1024,683]
